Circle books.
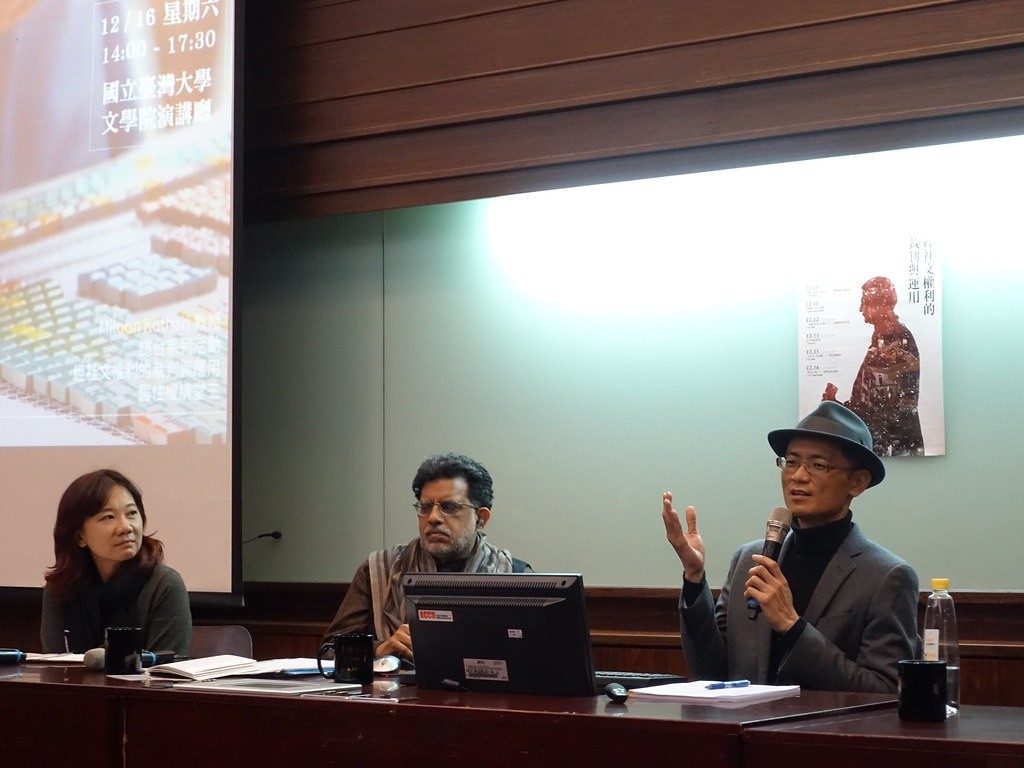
[145,655,257,682]
[628,680,800,701]
[230,658,335,678]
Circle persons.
[663,400,919,694]
[319,455,536,660]
[40,469,192,663]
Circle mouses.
[372,654,401,675]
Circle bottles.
[921,578,960,719]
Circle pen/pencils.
[705,680,751,690]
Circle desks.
[742,704,1024,768]
[122,675,899,768]
[0,661,193,768]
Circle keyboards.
[596,670,688,692]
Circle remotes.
[605,683,628,704]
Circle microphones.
[745,506,792,621]
[0,651,27,664]
[83,648,176,670]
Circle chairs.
[189,625,253,659]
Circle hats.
[768,401,887,488]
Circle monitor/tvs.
[401,571,597,697]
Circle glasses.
[776,457,857,475]
[413,499,480,517]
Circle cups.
[897,659,948,722]
[102,625,146,676]
[317,632,375,685]
